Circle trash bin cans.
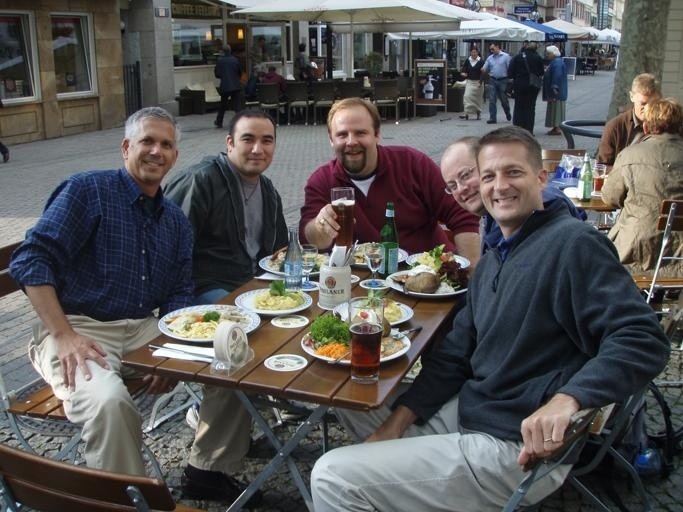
[446,86,464,113]
[178,88,206,115]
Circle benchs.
[174,79,222,117]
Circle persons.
[10,107,264,507]
[458,42,569,136]
[0,99,10,162]
[297,98,482,364]
[601,97,683,322]
[596,72,662,164]
[211,37,319,128]
[161,110,305,459]
[311,124,672,512]
[441,138,583,257]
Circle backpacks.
[606,380,683,486]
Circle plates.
[256,248,324,276]
[300,324,412,363]
[157,306,262,341]
[267,313,311,330]
[348,241,410,266]
[263,353,308,372]
[333,295,412,326]
[404,250,470,269]
[233,289,312,319]
[387,267,469,296]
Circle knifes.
[147,342,217,360]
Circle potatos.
[381,318,391,338]
[404,272,441,294]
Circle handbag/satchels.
[528,73,541,89]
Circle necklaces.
[237,173,260,206]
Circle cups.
[312,264,353,310]
[347,295,385,385]
[591,164,606,197]
[330,186,358,249]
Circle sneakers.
[547,129,561,135]
[185,403,199,431]
[280,400,305,420]
[459,115,468,120]
[486,120,497,124]
[477,118,481,120]
[505,113,511,121]
[213,120,223,128]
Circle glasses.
[444,164,477,195]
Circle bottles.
[577,150,593,203]
[379,201,401,279]
[283,227,303,291]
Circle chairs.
[0,441,197,511]
[540,147,585,182]
[631,198,683,338]
[501,311,675,512]
[215,64,476,127]
[0,242,180,482]
[576,48,618,77]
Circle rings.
[543,438,553,442]
[319,218,326,226]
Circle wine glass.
[300,245,318,289]
[358,243,389,290]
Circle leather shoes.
[180,470,263,507]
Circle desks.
[568,195,619,234]
